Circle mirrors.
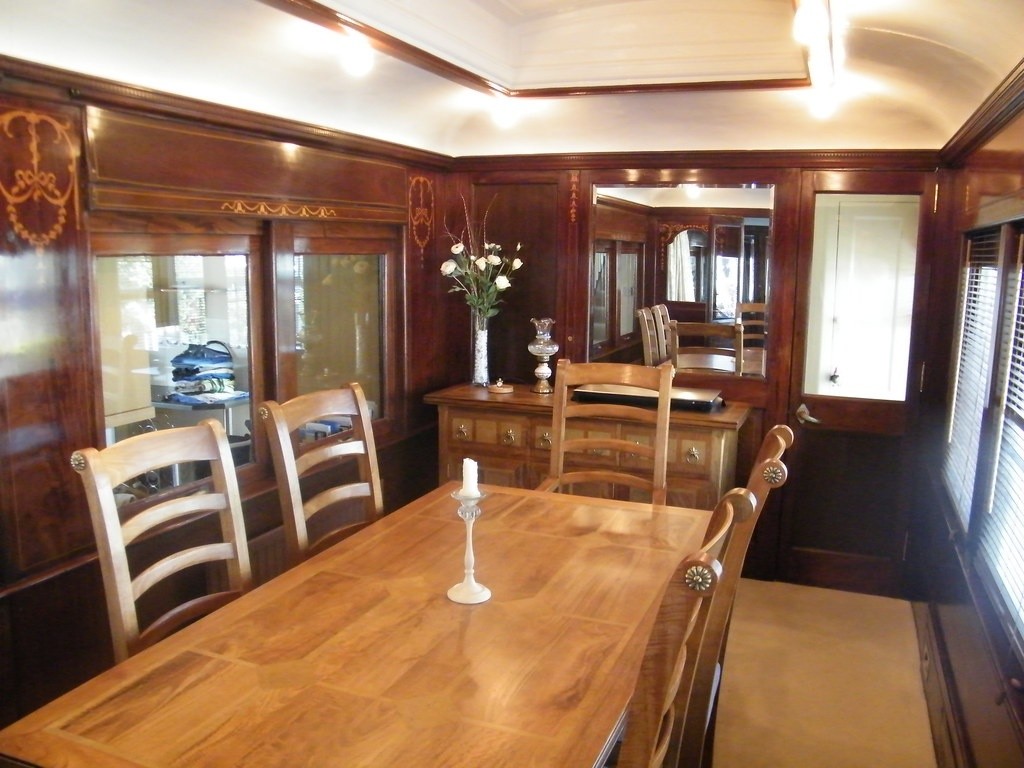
[576,169,800,380]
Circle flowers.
[440,189,524,319]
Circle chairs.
[678,423,794,768]
[633,302,765,377]
[607,485,757,768]
[534,357,675,508]
[71,418,254,664]
[264,379,385,565]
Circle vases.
[473,321,490,386]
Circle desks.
[151,384,249,437]
[0,479,714,768]
[654,345,762,375]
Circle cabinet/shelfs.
[421,385,750,511]
[93,256,157,429]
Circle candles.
[462,458,479,495]
[736,317,742,324]
